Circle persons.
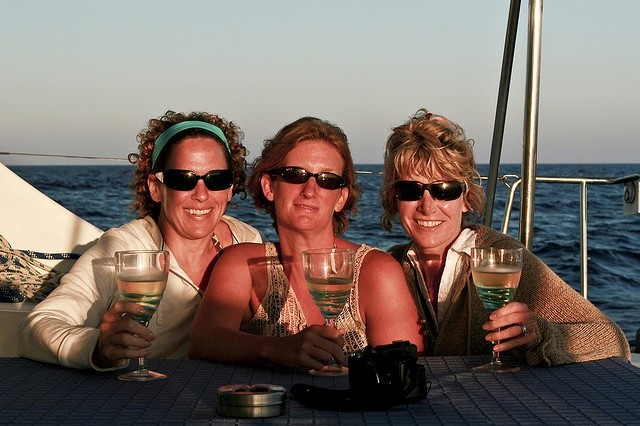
[187,116,425,371]
[379,108,631,367]
[16,110,250,372]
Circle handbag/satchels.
[0,234,82,305]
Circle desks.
[0,356,640,425]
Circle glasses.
[269,166,350,190]
[154,169,237,191]
[392,181,466,201]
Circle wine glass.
[301,247,356,377]
[113,249,170,382]
[470,246,524,378]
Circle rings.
[520,325,527,336]
